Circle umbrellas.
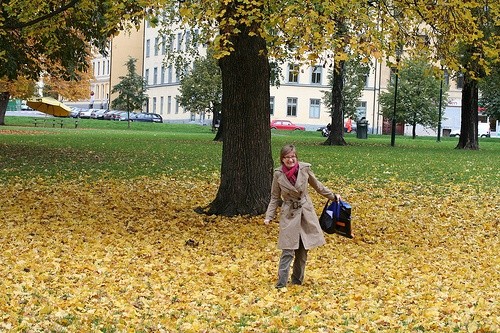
[26,95,71,128]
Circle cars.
[318,122,357,132]
[71,107,162,123]
[270,120,306,131]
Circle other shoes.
[275,284,284,289]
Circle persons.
[264,144,341,289]
[345,118,352,133]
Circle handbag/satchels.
[319,195,353,239]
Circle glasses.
[282,156,296,160]
